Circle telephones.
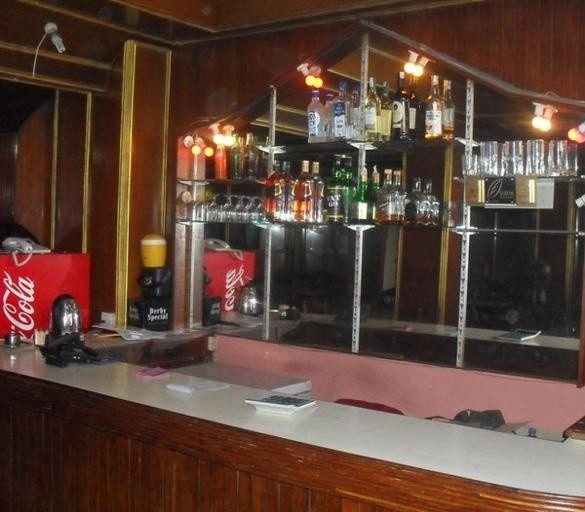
[334,308,370,323]
[36,342,101,368]
[1,237,52,254]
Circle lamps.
[310,64,324,89]
[564,120,585,138]
[543,104,556,131]
[414,55,430,76]
[530,100,547,130]
[295,61,316,87]
[196,134,215,158]
[402,47,420,75]
[222,124,237,148]
[31,20,68,77]
[181,135,203,157]
[206,122,226,145]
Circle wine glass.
[193,190,263,224]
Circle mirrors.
[177,40,585,388]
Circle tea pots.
[237,283,262,315]
[47,295,85,344]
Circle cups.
[461,136,581,178]
[33,328,47,346]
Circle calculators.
[244,395,317,416]
[496,328,541,342]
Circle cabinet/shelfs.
[262,132,457,229]
[452,173,584,239]
[174,173,274,228]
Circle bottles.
[139,233,167,267]
[305,69,455,143]
[213,128,269,179]
[262,154,441,229]
